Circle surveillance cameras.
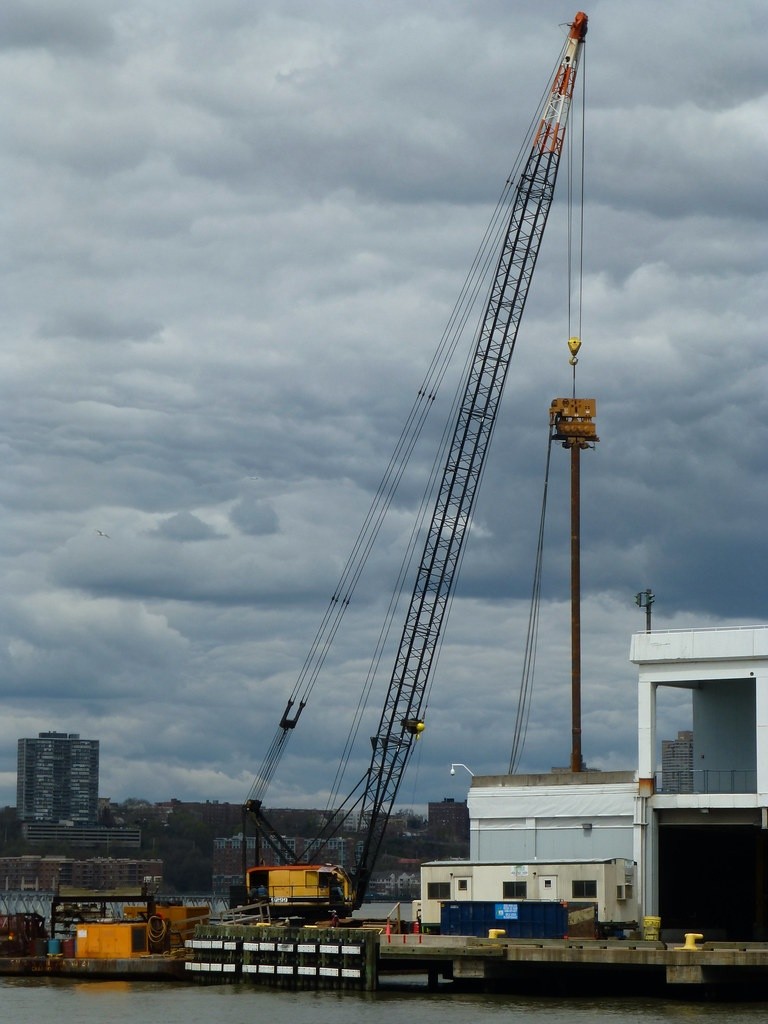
[450,769,455,776]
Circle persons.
[329,873,345,897]
[247,884,268,903]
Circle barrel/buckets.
[63,939,75,958]
[644,916,661,941]
[35,939,47,956]
[48,939,60,955]
